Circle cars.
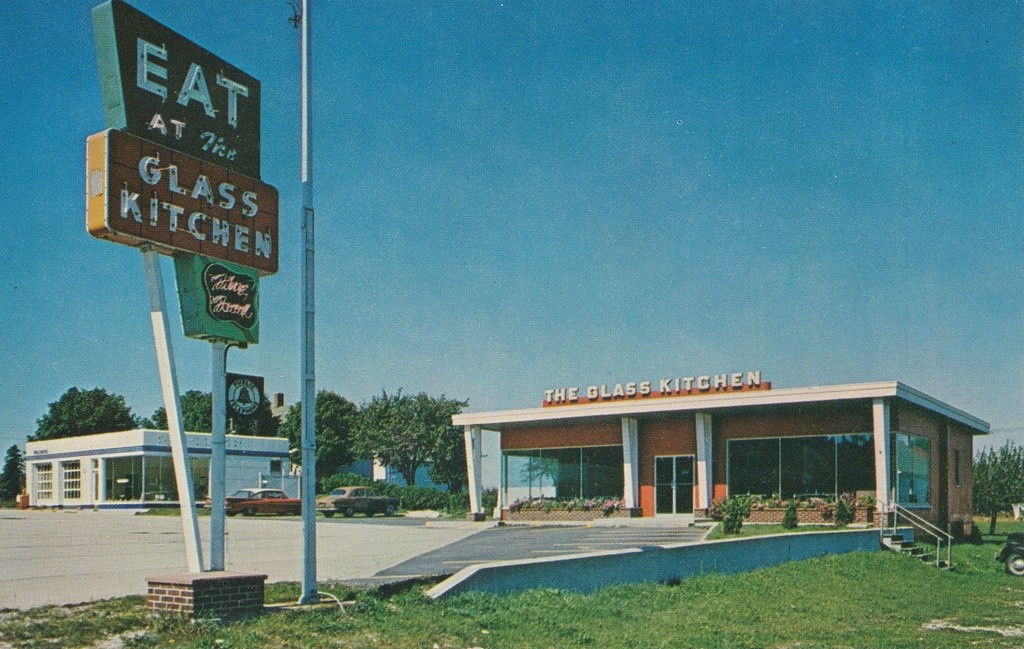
[224,488,301,517]
[315,486,399,518]
[996,531,1024,576]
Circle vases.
[502,508,643,523]
[714,508,877,523]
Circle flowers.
[506,495,623,518]
[707,493,874,509]
